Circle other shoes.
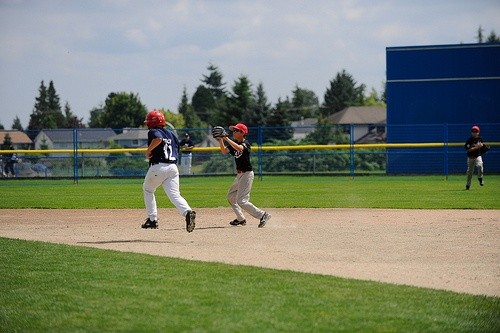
[465,185,470,191]
[478,178,484,186]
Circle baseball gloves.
[212,126,228,138]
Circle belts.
[237,170,245,173]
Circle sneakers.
[230,219,246,226]
[141,218,158,229]
[258,212,272,228]
[186,211,196,232]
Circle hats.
[229,124,248,134]
[472,126,480,132]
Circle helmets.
[146,111,165,128]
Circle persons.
[212,122,271,228]
[179,131,195,176]
[464,125,489,190]
[3,142,18,178]
[141,109,197,233]
